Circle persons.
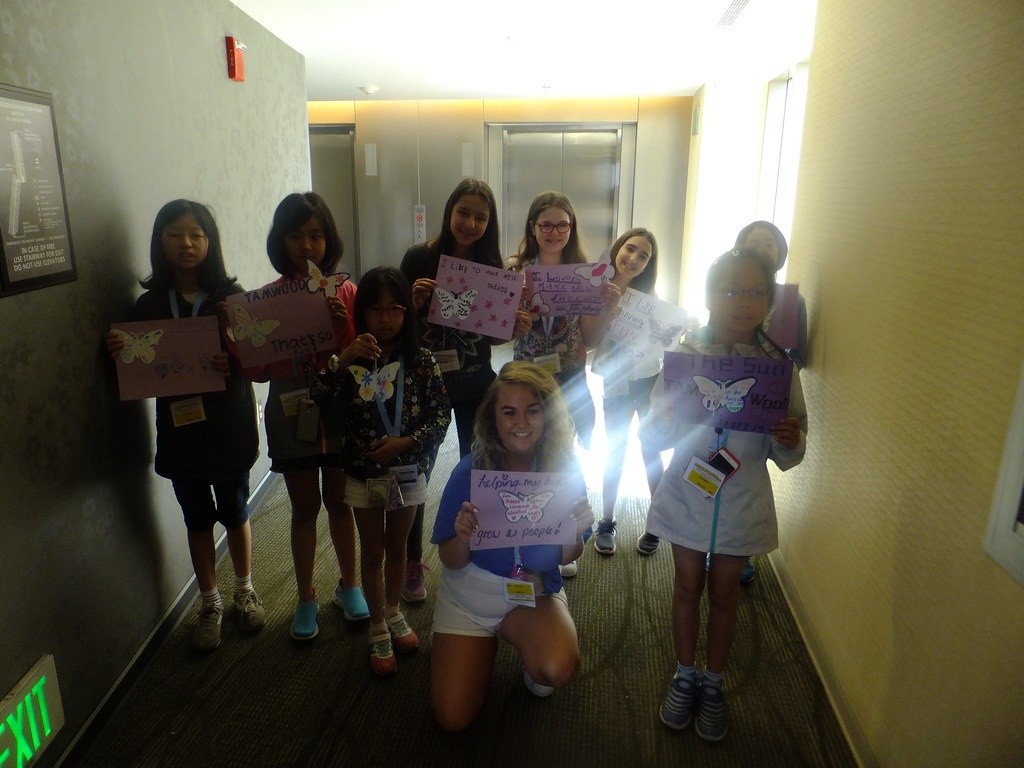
[706,221,808,581]
[310,266,451,675]
[640,249,808,741]
[216,192,371,639]
[591,227,700,555]
[504,191,620,577]
[429,360,595,734]
[400,179,532,602]
[105,198,267,649]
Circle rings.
[521,321,524,327]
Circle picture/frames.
[0,81,78,296]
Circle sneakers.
[558,560,577,577]
[401,558,430,602]
[637,531,660,554]
[695,674,728,741]
[367,629,397,676]
[192,603,224,650]
[289,588,320,640]
[333,582,371,622]
[658,668,700,729]
[739,562,755,582]
[383,606,420,652]
[232,589,266,631]
[593,519,617,554]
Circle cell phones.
[296,398,320,445]
[702,448,740,499]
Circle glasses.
[718,287,769,301]
[534,221,572,233]
[367,303,406,317]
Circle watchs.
[328,355,343,374]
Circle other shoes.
[523,669,554,698]
[429,632,434,647]
